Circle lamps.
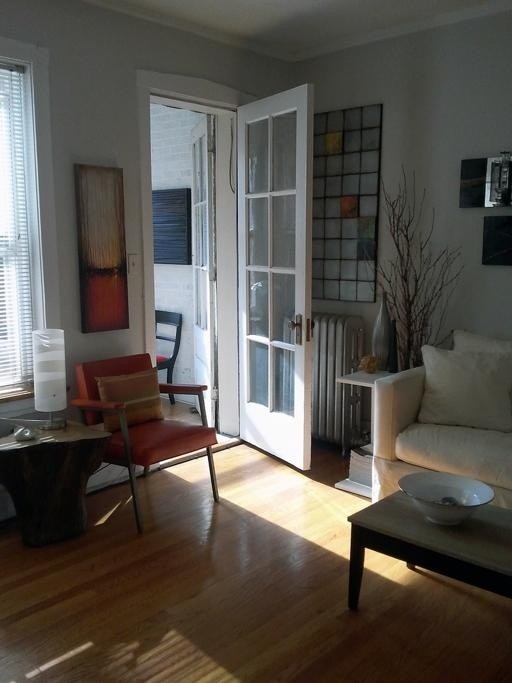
[32,328,67,430]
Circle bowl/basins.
[396,470,496,526]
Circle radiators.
[278,311,365,459]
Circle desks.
[347,490,512,610]
[0,419,112,548]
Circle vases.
[371,292,392,370]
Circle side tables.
[334,371,395,499]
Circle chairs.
[70,353,220,535]
[155,310,182,404]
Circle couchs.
[371,365,512,505]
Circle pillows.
[95,368,164,432]
[418,345,512,433]
[452,330,512,350]
